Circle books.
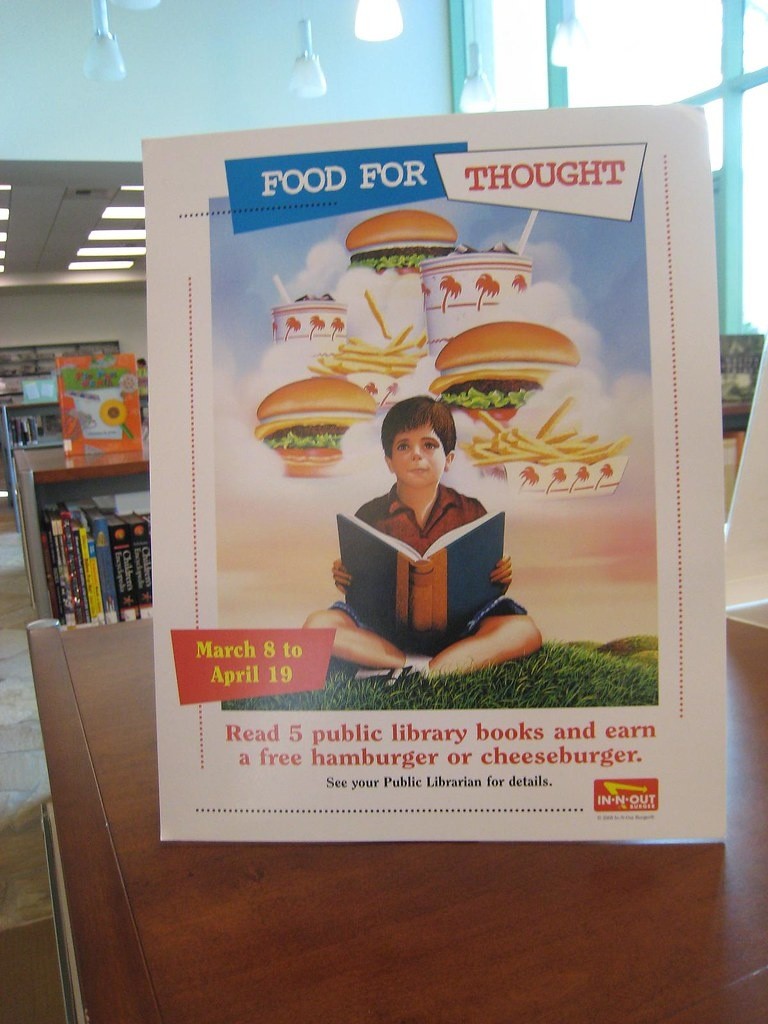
[40,500,152,626]
[718,333,765,536]
[55,353,144,460]
[8,410,44,447]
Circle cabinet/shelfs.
[0,341,768,1024]
